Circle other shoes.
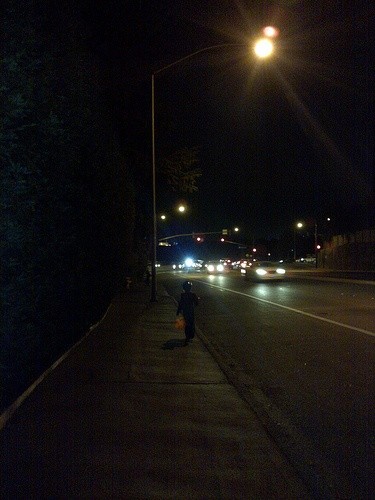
[185,337,192,342]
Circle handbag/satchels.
[176,316,185,334]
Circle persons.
[176,280,200,345]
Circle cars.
[172,257,252,275]
[245,261,287,282]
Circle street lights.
[151,38,276,303]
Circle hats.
[183,281,192,289]
